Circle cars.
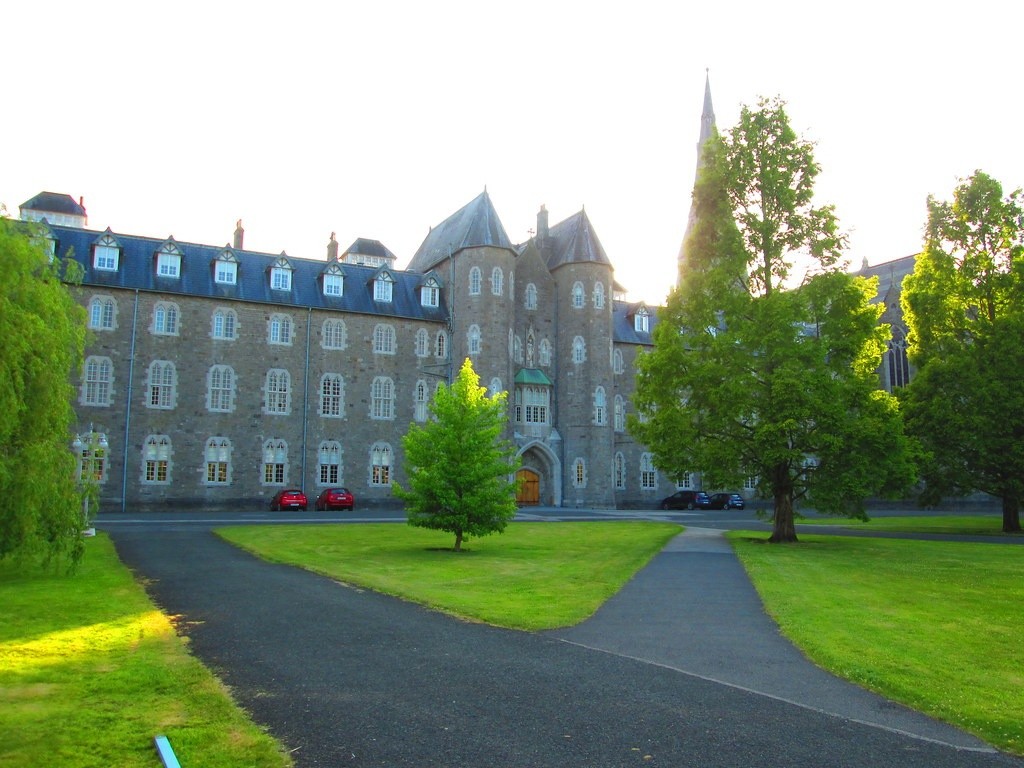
[270,488,308,511]
[314,487,354,511]
[661,489,713,511]
[708,493,746,511]
